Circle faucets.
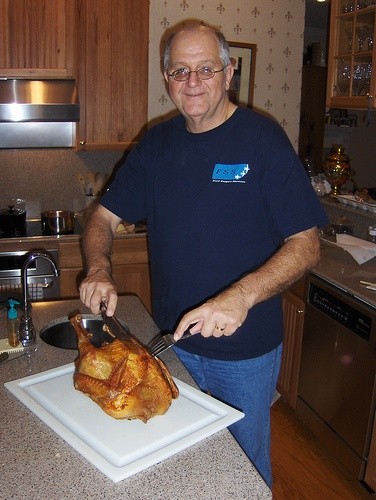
[21,253,59,347]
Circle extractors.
[0,79,80,148]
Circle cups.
[85,196,95,208]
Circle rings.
[215,326,225,332]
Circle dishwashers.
[295,273,376,481]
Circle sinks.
[39,314,130,350]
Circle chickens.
[65,301,180,425]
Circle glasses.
[167,64,228,82]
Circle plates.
[337,195,376,214]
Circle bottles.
[366,225,376,243]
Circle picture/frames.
[226,41,257,110]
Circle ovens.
[0,249,60,305]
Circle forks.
[147,329,201,358]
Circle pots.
[40,207,88,235]
[0,204,27,236]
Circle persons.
[79,26,328,486]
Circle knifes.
[100,302,142,356]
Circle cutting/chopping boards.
[3,359,246,481]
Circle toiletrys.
[8,300,20,348]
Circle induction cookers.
[0,217,84,238]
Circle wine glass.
[337,64,371,96]
[343,26,374,54]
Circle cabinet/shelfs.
[276,290,305,408]
[325,0,376,110]
[74,0,148,149]
[0,0,77,77]
[57,241,153,316]
